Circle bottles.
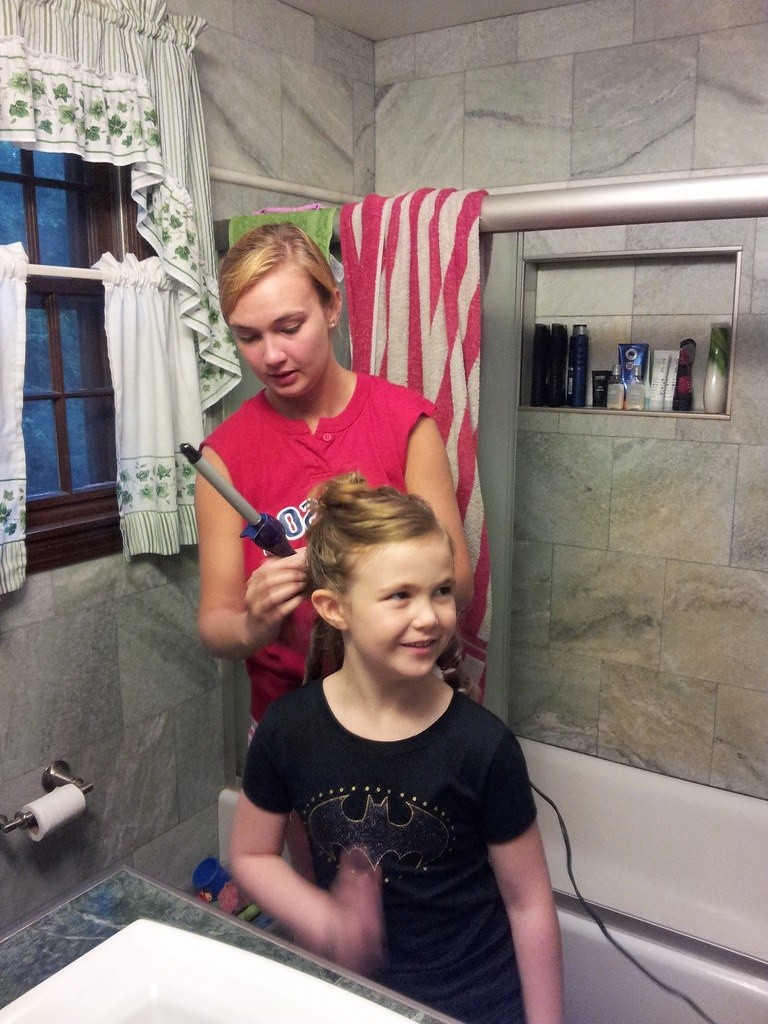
[625,365,649,407]
[605,364,625,410]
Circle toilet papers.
[20,782,87,843]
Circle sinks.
[1,915,422,1024]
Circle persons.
[230,477,569,1024]
[194,224,473,748]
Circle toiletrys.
[530,321,730,415]
